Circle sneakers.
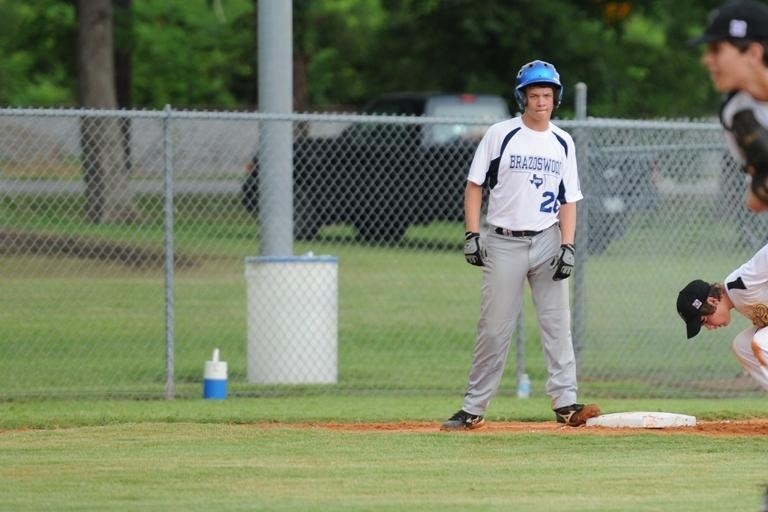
[556,402,600,426]
[443,410,485,429]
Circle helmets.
[513,59,563,107]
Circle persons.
[674,240,768,392]
[438,56,603,429]
[686,0,768,213]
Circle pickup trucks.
[242,92,658,259]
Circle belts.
[494,227,544,237]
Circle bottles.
[517,373,529,399]
[202,348,228,400]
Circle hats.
[676,279,711,339]
[694,1,768,44]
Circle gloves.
[549,245,575,281]
[464,230,487,267]
